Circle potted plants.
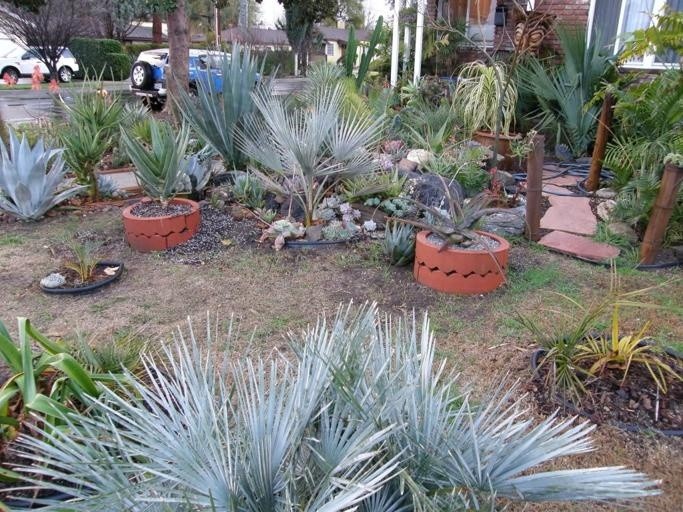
[4,296,663,510]
[1,3,682,254]
[40,232,125,295]
[363,170,533,295]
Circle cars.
[0,46,79,83]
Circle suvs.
[131,48,263,106]
